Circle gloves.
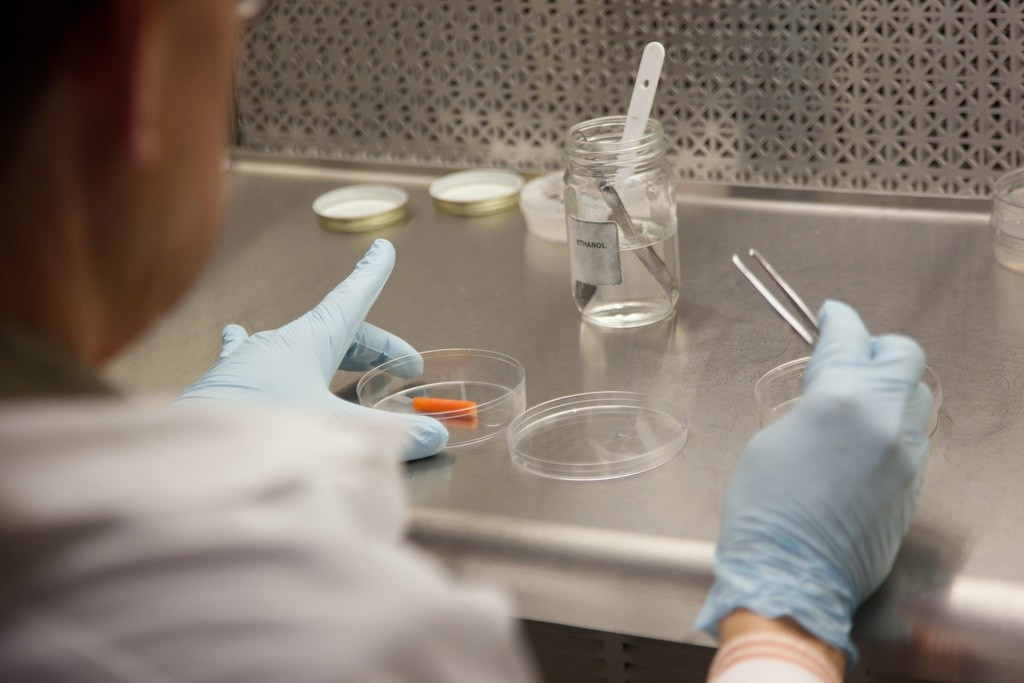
[694,300,934,672]
[165,238,448,462]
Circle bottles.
[567,117,680,328]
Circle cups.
[989,168,1024,274]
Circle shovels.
[574,41,664,311]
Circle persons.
[0,0,939,683]
